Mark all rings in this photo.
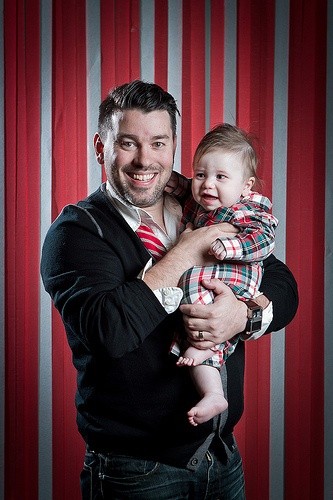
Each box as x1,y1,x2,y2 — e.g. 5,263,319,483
198,331,204,341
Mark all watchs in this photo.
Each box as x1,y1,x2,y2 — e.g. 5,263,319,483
243,301,262,334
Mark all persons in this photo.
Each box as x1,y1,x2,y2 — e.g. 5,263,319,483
164,123,280,426
40,80,298,500
